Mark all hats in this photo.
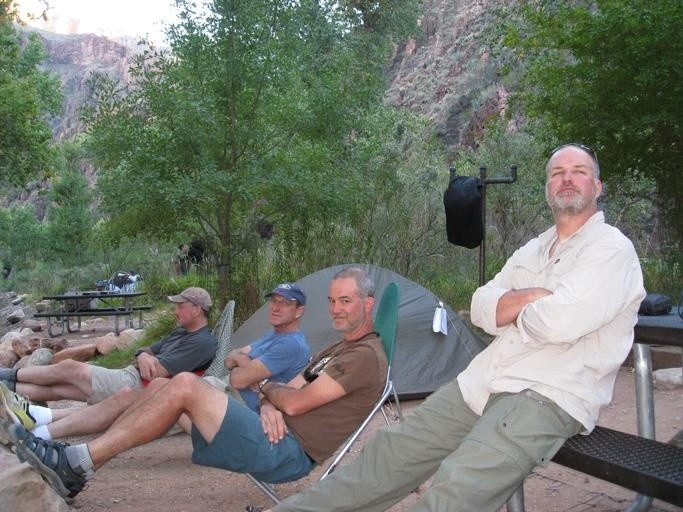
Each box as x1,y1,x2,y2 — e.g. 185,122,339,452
166,287,213,312
264,282,307,305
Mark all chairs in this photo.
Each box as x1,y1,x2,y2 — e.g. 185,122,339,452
243,282,404,504
200,299,235,380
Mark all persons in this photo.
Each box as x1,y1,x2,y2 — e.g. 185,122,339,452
1,286,219,408
127,270,141,290
174,239,204,277
260,144,648,512
0,281,311,443
8,268,387,506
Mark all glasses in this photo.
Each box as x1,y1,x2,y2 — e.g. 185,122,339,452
303,357,330,382
550,141,597,156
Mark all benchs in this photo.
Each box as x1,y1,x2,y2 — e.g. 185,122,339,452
32,305,152,338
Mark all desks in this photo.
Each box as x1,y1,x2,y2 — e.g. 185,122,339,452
41,291,146,311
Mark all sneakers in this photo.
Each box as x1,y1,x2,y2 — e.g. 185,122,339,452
0,382,37,433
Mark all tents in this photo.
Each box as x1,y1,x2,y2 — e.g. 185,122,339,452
231,263,490,403
110,270,131,291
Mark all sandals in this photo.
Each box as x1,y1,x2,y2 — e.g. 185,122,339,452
9,423,88,499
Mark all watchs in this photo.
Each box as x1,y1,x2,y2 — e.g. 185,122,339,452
257,378,271,390
